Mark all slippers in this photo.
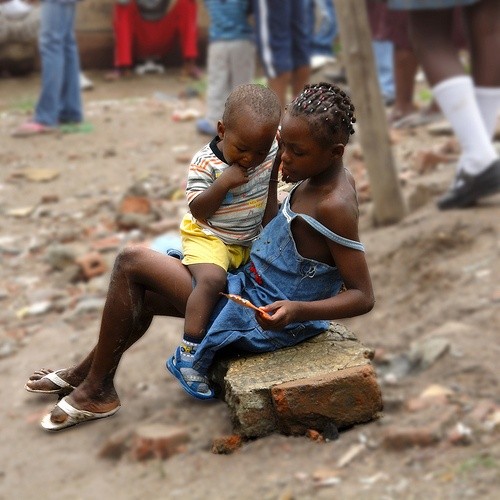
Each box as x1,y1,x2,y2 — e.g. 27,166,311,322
24,369,78,394
40,396,120,432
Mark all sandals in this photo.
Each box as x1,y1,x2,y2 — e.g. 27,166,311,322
167,346,214,399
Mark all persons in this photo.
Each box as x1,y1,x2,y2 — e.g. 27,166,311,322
389,0,500,215
381,0,456,128
308,1,336,70
23,83,376,431
253,0,311,130
167,85,282,399
17,0,82,137
366,1,396,104
107,0,202,84
0,0,94,92
197,0,255,134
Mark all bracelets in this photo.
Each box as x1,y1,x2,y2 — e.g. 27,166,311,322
269,178,279,184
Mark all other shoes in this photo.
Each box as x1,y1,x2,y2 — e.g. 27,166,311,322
79,73,93,90
15,123,55,137
325,68,347,86
435,160,500,210
197,121,214,134
